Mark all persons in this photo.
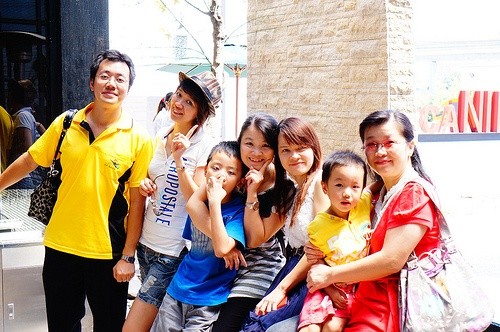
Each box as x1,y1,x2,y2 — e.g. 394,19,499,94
304,109,442,332
153,92,175,139
148,140,252,332
0,105,14,176
7,79,42,189
120,70,222,332
297,150,386,332
186,114,296,332
0,50,154,332
239,116,331,332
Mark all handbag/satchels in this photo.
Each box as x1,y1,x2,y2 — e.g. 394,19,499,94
396,250,496,332
27,109,78,225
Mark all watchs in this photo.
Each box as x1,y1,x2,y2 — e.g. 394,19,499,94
121,255,135,263
245,201,260,211
176,165,186,170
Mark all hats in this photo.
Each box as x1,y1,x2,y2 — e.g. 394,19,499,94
178,70,222,117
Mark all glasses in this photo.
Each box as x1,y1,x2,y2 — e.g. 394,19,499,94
360,141,398,153
97,73,126,85
149,175,174,216
